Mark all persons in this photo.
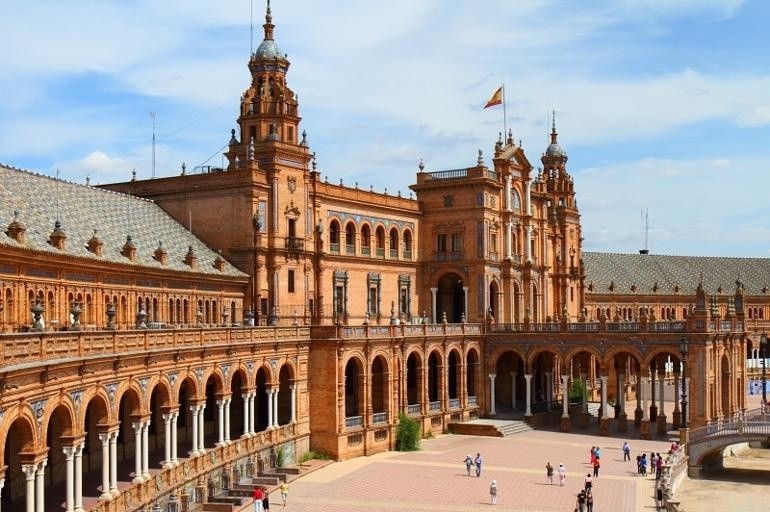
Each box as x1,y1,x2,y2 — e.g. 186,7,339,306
545,442,679,512
262,485,270,512
474,452,481,476
489,479,497,504
253,486,264,511
463,454,473,475
280,481,289,505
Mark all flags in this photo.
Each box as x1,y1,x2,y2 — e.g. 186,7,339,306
481,86,501,111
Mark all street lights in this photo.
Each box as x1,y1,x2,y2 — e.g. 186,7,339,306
677,336,690,445
760,328,768,400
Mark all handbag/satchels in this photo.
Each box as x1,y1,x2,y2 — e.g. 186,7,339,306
574,508,578,512
